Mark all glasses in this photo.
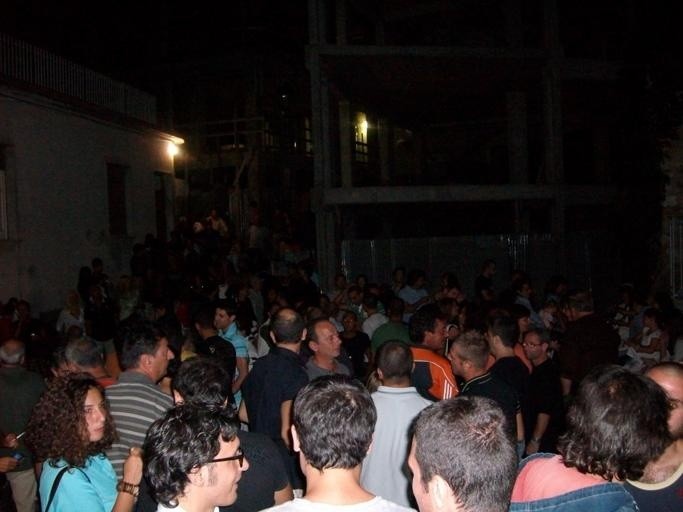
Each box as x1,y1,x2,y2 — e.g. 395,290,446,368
522,341,543,348
205,446,245,468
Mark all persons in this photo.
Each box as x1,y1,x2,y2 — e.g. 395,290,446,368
1,208,683,512
